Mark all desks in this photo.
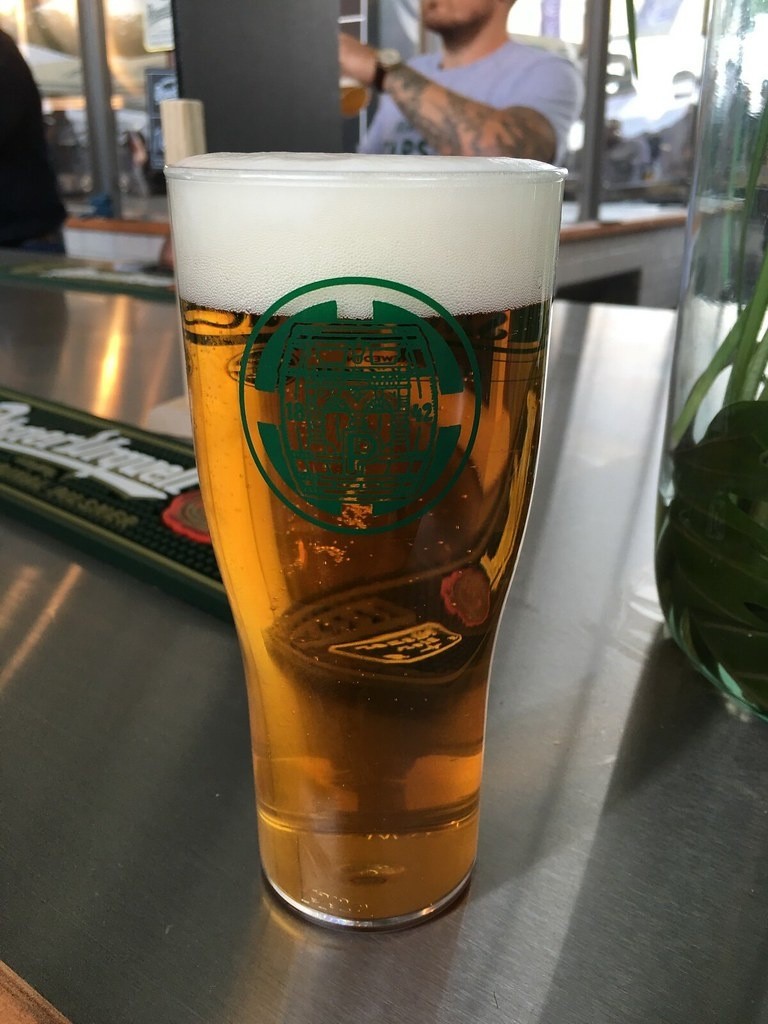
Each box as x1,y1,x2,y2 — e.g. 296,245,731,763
0,268,768,1022
58,192,693,301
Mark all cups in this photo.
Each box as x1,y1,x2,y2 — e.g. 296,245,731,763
166,143,565,935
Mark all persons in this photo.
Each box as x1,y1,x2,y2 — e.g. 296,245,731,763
338,0,588,168
0,29,73,260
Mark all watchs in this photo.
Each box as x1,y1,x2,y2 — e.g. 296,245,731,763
369,47,403,93
